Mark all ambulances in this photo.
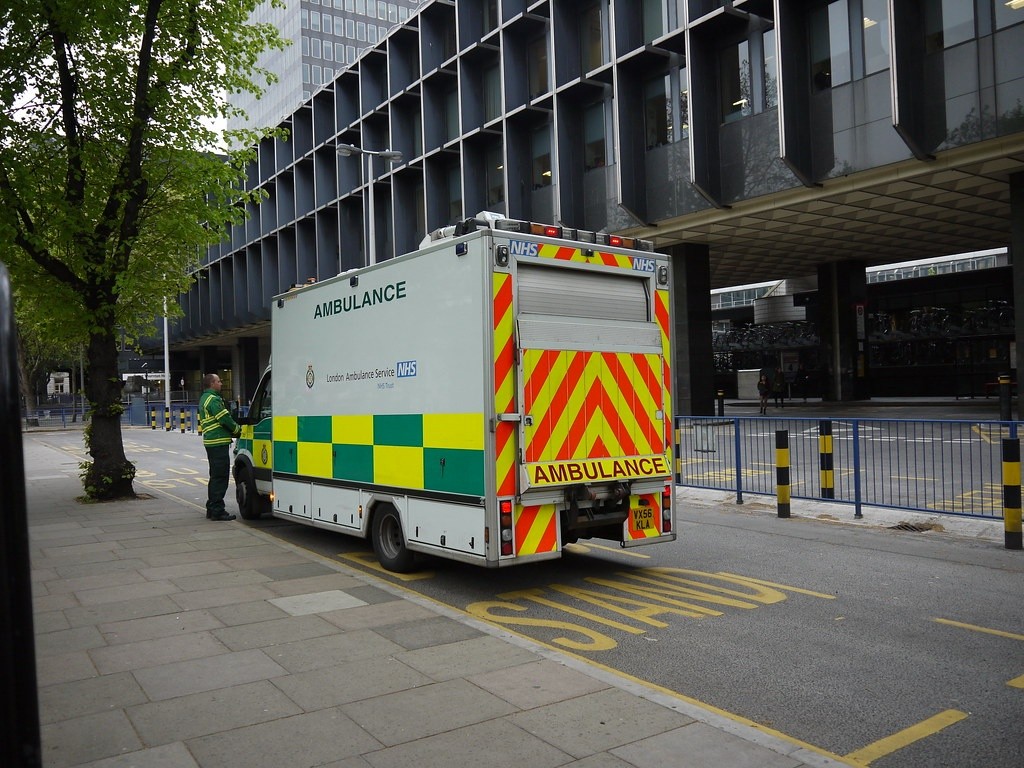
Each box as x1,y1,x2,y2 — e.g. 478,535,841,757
231,210,682,575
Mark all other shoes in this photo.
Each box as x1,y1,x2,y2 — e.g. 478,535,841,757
206,509,236,520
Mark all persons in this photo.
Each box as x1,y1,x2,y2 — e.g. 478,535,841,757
199,374,242,521
772,377,785,408
757,375,770,415
